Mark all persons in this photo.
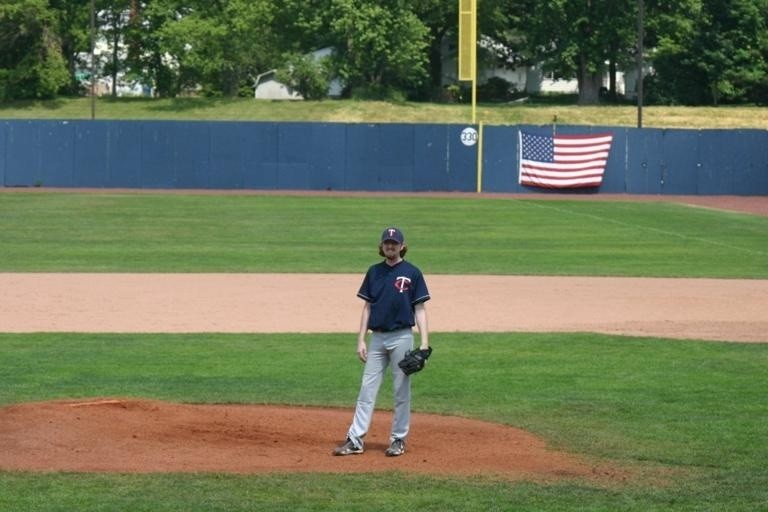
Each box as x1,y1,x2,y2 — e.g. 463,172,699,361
330,227,431,456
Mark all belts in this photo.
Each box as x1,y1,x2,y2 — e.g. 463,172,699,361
371,325,413,333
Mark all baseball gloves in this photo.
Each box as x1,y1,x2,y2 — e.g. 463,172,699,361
398,346,432,375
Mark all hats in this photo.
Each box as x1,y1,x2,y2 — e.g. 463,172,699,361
380,227,405,244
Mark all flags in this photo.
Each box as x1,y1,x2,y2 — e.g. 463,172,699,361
518,129,612,189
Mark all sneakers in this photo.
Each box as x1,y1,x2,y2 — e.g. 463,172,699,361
332,436,366,456
385,439,406,456
